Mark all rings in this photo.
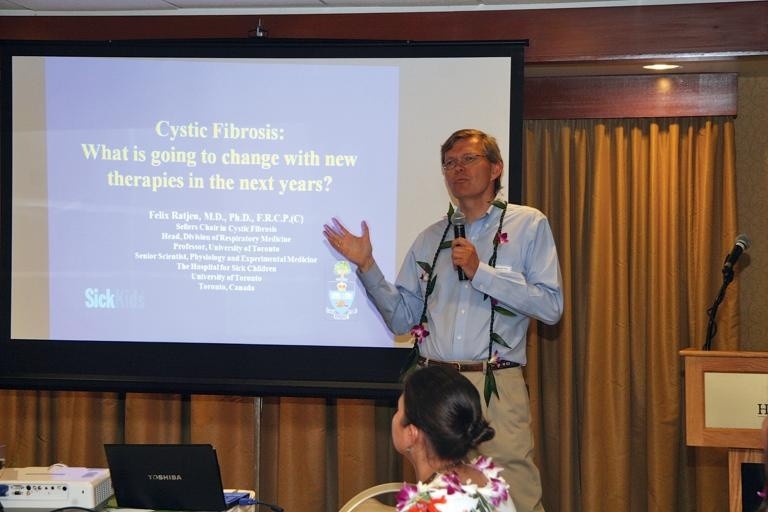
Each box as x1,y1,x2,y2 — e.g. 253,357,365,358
337,241,341,248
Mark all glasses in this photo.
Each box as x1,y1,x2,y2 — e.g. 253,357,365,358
441,154,487,170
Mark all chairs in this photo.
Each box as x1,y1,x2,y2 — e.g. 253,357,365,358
340,481,425,512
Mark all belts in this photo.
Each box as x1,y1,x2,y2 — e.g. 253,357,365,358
417,353,520,372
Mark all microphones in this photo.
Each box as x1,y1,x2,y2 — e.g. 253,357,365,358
723,232,751,274
451,211,470,280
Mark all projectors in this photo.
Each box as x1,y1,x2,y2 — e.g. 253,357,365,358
0,462,112,511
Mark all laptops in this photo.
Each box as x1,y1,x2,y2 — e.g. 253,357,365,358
105,440,250,511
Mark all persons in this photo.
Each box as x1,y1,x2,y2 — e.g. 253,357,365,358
390,363,518,512
321,127,565,512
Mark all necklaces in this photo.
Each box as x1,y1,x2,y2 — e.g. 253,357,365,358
423,461,461,484
